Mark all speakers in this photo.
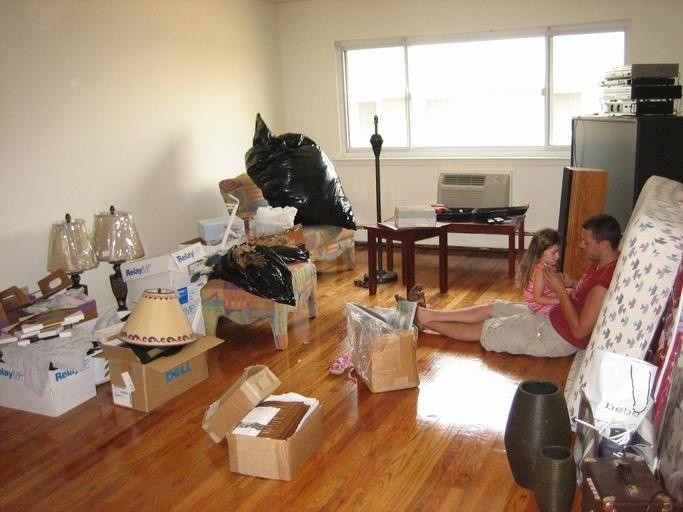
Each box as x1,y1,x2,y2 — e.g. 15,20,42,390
558,166,608,280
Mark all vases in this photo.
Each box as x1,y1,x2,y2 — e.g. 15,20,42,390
503,375,573,492
531,440,577,512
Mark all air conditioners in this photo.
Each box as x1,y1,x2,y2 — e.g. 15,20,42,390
436,172,511,209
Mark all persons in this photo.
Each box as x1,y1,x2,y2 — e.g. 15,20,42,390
513,227,578,313
393,213,620,359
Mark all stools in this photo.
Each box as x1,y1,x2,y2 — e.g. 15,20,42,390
194,261,318,349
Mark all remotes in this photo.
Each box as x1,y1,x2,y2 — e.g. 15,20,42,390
494,217,504,223
486,217,495,224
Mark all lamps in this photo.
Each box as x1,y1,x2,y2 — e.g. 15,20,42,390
90,204,146,313
42,211,99,294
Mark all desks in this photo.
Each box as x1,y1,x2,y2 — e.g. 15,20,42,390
383,208,526,279
362,220,453,299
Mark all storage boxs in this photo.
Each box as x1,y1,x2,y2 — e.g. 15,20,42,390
2,244,226,419
248,224,304,248
335,294,420,393
197,215,245,241
199,364,283,445
224,396,326,484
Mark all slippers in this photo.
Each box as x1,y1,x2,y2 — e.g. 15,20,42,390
393,294,427,333
408,283,427,310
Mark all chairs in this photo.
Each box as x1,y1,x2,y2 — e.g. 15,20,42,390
217,169,358,271
